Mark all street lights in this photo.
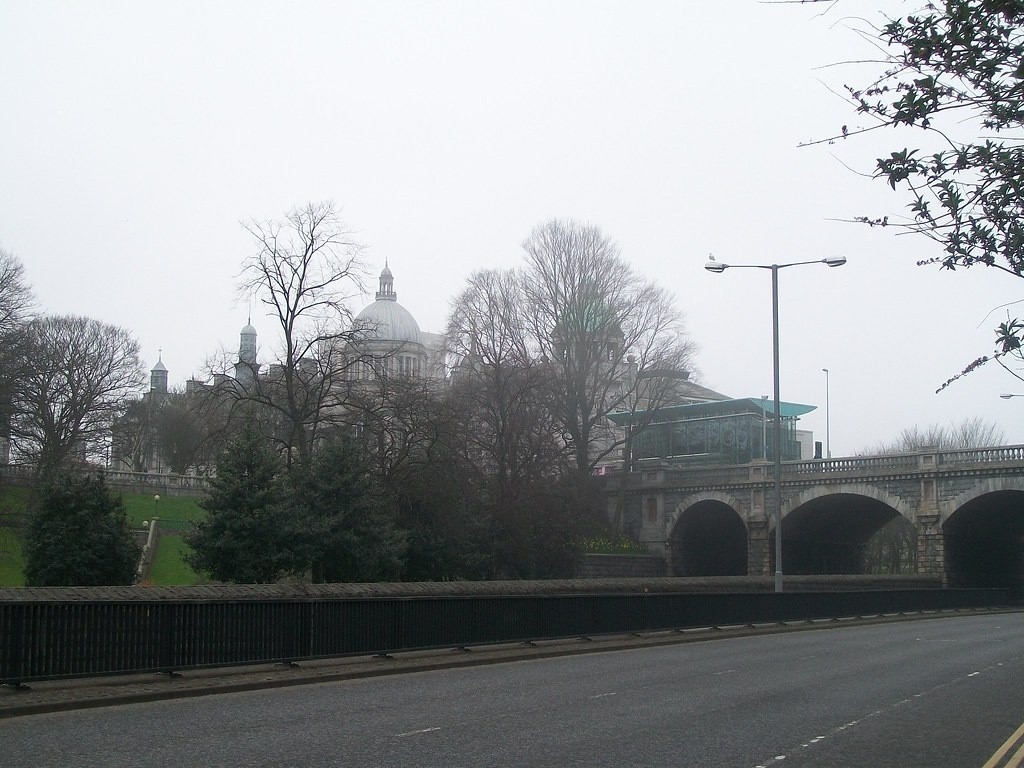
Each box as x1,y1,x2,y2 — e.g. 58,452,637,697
821,368,830,458
760,395,768,460
154,495,160,517
143,520,148,545
705,256,848,590
